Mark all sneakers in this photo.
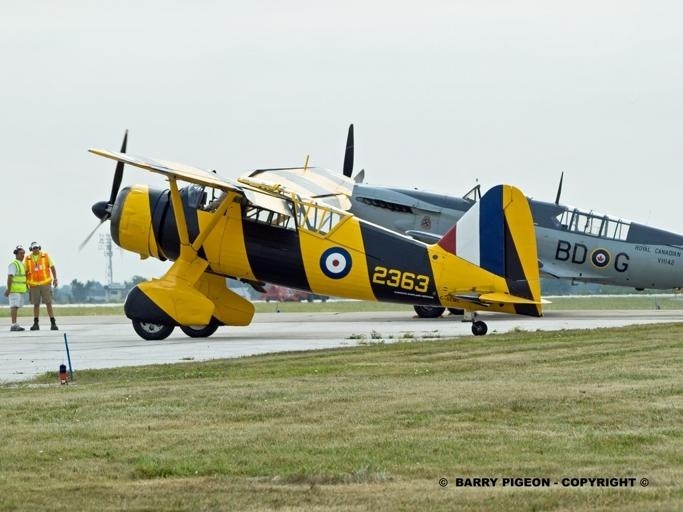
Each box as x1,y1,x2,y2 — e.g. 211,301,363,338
10,324,25,331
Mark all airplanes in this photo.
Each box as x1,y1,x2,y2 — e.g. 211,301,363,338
76,123,683,340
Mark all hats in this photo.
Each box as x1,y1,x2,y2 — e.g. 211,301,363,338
31,242,40,248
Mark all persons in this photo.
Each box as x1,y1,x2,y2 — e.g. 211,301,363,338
3,242,59,331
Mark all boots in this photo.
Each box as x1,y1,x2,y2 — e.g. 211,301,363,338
29,318,40,330
49,317,59,330
